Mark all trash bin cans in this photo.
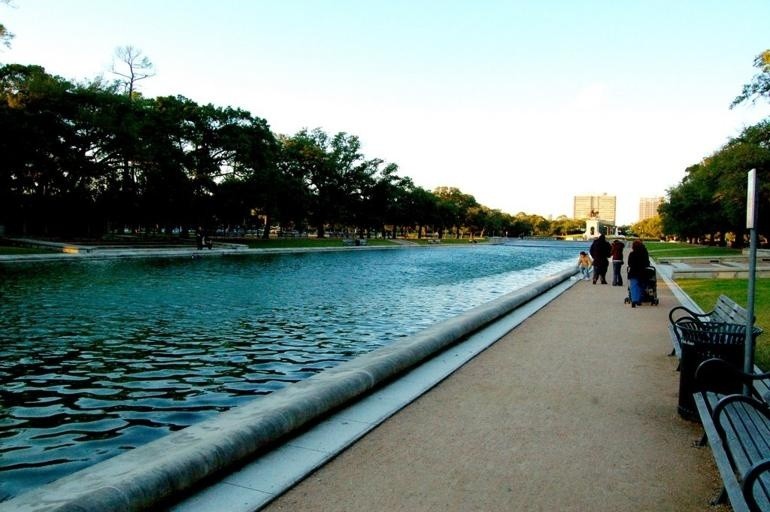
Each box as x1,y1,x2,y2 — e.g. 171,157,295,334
674,320,764,423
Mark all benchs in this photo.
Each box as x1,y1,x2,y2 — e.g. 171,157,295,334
667,291,770,511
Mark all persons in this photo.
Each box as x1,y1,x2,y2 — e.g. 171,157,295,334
590,235,611,284
196,224,206,249
626,240,649,307
612,239,624,287
577,252,593,281
714,231,767,248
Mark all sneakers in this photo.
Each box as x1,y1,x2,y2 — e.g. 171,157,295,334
618,282,622,285
612,282,617,286
632,301,641,308
593,278,597,284
601,281,607,284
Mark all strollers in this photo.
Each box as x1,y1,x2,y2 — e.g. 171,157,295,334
624,266,658,306
